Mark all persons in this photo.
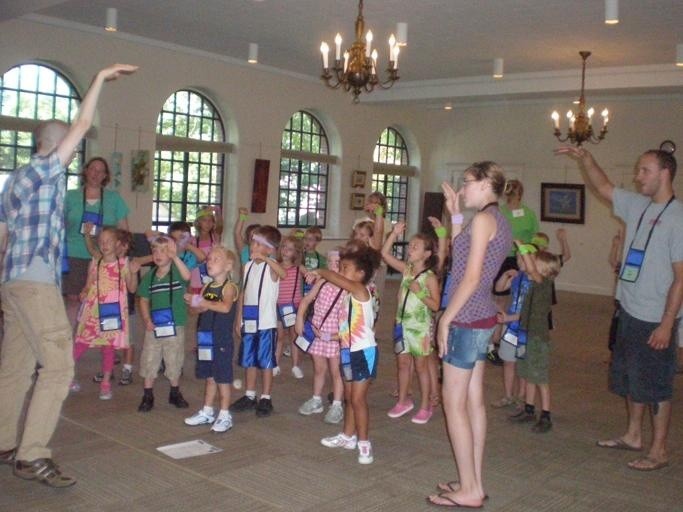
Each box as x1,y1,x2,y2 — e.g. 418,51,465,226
0,63,139,488
426,160,519,511
554,146,683,470
132,178,571,465
63,157,133,402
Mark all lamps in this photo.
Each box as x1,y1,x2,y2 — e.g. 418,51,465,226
491,58,504,79
676,44,682,66
104,7,117,31
246,43,257,64
549,51,611,146
318,1,403,105
394,23,407,48
603,0,618,25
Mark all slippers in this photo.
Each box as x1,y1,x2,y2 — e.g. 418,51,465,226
437,480,490,505
425,492,482,512
628,455,669,472
596,437,644,452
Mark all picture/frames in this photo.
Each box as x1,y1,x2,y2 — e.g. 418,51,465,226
540,182,584,225
351,170,366,188
351,192,364,209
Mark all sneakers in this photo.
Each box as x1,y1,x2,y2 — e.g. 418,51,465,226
13,457,74,488
93,355,190,412
298,386,442,465
492,394,553,432
185,367,303,433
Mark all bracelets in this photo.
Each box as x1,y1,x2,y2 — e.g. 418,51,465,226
451,213,464,226
